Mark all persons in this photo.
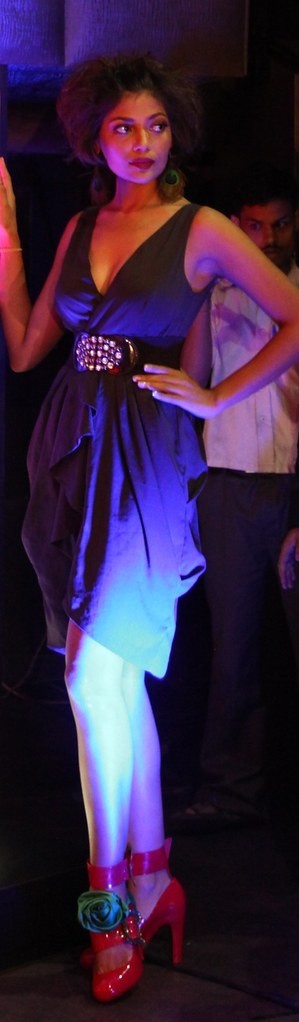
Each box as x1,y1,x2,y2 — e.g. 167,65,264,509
0,50,299,998
169,173,299,828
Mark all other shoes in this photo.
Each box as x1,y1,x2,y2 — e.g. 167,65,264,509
170,802,260,829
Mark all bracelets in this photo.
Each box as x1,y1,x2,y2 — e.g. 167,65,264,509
0,248,23,251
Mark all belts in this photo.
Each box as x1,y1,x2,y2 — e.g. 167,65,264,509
70,331,181,376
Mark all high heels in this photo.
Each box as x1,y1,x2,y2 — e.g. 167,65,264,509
80,837,186,972
86,854,144,1002
